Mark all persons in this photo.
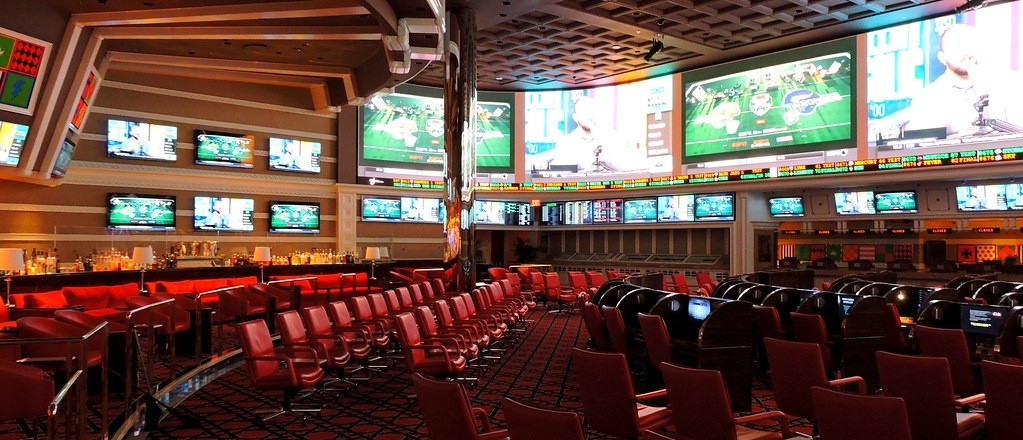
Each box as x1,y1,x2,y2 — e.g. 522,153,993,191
546,95,622,173
964,188,987,209
842,194,860,213
205,200,230,228
121,125,151,156
476,203,492,222
662,198,680,220
407,200,424,221
280,142,301,169
906,23,1023,137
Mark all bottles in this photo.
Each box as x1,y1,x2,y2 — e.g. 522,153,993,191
22,247,360,277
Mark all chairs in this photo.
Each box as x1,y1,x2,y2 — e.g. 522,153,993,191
0,262,1023,439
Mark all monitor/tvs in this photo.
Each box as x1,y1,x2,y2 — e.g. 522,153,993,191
833,191,919,215
106,119,178,163
105,192,176,233
688,298,712,321
959,303,1009,338
193,129,255,169
268,137,322,174
956,183,1023,212
193,197,255,233
769,197,804,217
359,193,534,227
539,191,736,227
268,200,321,234
841,294,863,314
